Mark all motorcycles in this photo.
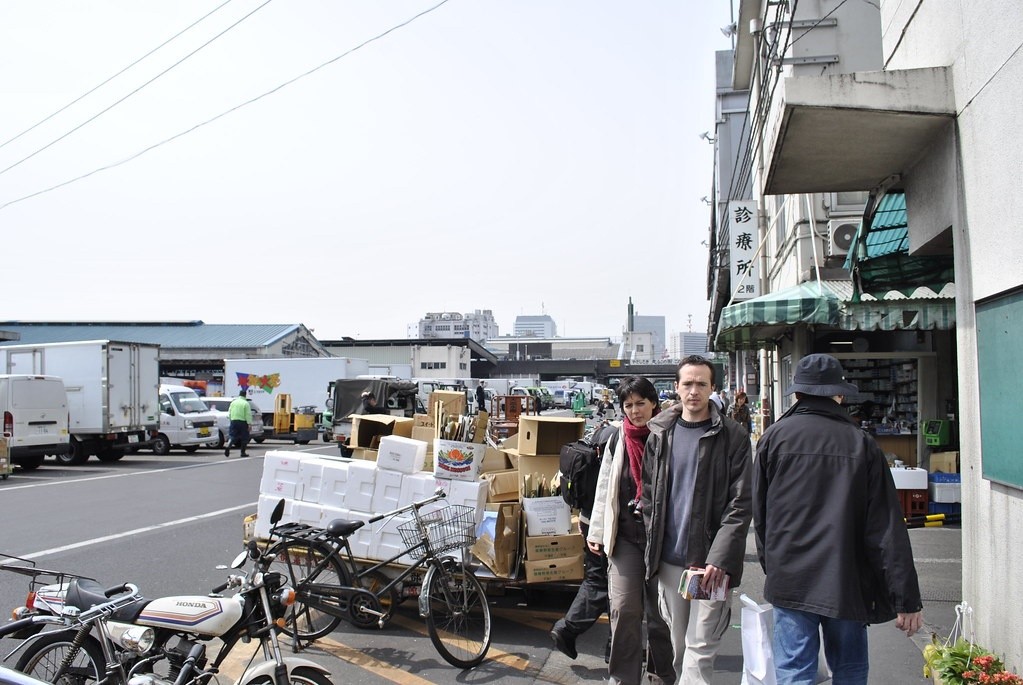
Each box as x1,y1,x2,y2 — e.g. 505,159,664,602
488,396,540,445
0,497,342,685
597,409,617,420
239,514,732,640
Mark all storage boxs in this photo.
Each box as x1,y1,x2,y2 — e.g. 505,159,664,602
890,451,961,529
0,437,9,474
255,389,587,584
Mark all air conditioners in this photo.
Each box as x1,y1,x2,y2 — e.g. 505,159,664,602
828,219,862,256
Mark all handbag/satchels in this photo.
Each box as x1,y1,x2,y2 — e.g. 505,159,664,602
740,593,833,685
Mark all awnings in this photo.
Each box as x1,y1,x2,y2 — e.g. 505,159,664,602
714,281,956,353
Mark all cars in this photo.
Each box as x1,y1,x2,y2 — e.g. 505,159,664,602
658,390,674,401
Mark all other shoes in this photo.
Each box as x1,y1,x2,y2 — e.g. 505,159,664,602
605,640,646,663
552,629,578,660
241,453,249,457
225,447,230,457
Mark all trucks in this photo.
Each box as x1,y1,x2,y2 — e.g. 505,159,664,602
222,359,618,458
0,340,161,465
0,374,70,472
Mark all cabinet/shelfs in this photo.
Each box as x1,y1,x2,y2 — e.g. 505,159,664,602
839,359,918,435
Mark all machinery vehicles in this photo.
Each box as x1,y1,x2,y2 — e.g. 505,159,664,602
253,394,319,446
322,381,336,442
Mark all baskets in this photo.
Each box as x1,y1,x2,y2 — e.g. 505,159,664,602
396,504,476,560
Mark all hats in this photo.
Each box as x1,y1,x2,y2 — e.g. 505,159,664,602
783,354,858,397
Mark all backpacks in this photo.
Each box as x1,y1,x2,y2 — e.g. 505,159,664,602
560,425,613,509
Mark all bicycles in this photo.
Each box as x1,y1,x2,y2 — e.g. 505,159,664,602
0,581,143,685
255,487,491,669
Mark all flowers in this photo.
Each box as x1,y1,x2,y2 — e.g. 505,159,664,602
922,631,1023,685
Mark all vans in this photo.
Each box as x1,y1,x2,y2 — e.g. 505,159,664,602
162,398,266,449
127,384,220,455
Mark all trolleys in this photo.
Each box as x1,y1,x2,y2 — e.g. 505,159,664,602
574,407,594,420
0,432,11,479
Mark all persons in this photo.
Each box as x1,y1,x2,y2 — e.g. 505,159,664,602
586,378,675,685
361,391,386,414
224,390,252,457
576,388,586,410
476,381,485,411
709,385,752,438
640,355,753,685
536,394,542,416
752,353,923,685
551,419,647,663
597,395,617,420
